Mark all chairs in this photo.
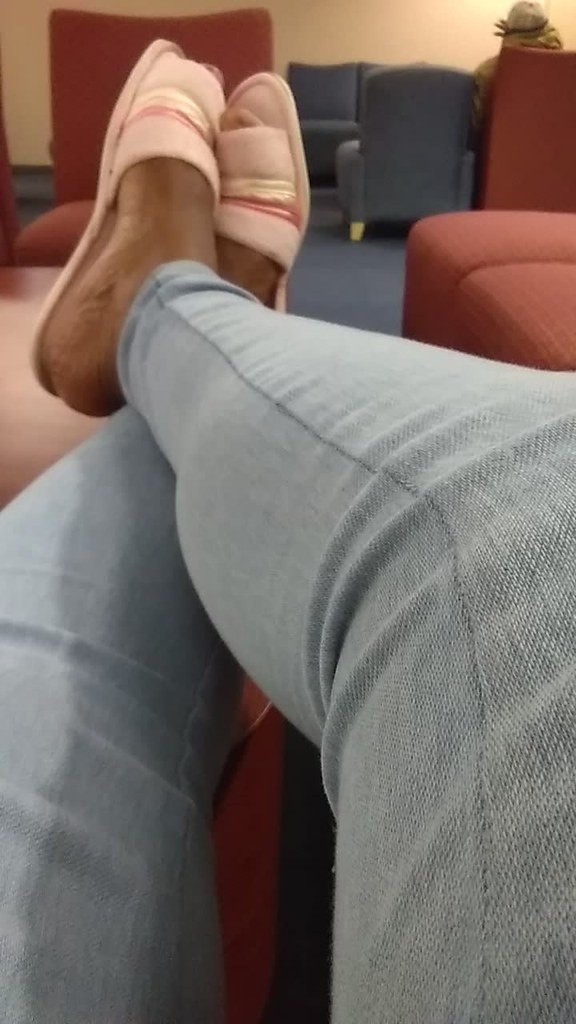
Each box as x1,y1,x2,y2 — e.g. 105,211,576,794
0,9,576,1024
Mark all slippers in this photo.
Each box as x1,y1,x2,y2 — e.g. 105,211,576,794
30,39,309,396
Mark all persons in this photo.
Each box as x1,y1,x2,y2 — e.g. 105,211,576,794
471,1,567,124
0,38,576,1024
